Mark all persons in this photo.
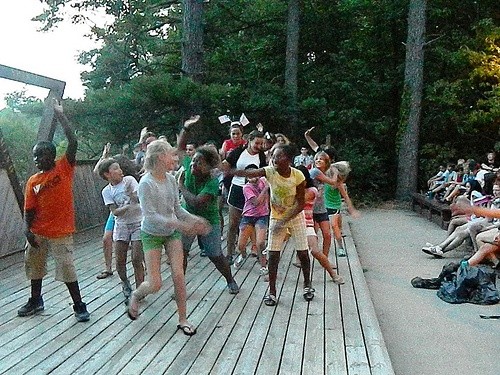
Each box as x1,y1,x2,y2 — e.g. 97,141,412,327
422,143,500,269
128,140,210,336
93,115,360,307
17,98,91,323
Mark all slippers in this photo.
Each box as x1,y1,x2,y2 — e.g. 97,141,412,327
128,290,138,320
96,271,113,279
177,324,196,335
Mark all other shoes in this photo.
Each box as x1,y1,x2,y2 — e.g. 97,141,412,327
73,302,90,322
234,252,248,269
260,267,268,276
263,291,277,306
421,243,443,258
122,280,131,297
18,295,44,316
338,248,346,257
330,276,345,284
227,280,239,294
200,250,206,257
303,286,315,301
225,255,234,265
292,263,302,268
